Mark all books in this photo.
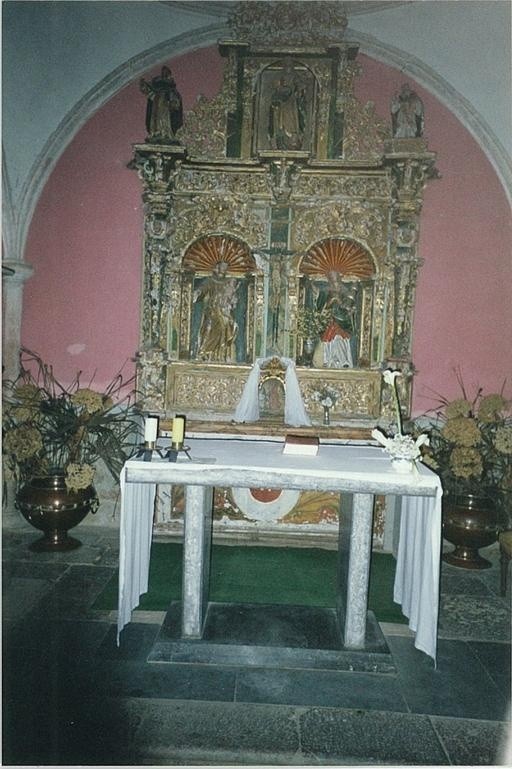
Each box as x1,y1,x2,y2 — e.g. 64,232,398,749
283,435,319,455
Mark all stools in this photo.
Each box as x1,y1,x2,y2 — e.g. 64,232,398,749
495,526,512,597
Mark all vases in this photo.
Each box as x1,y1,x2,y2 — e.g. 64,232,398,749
388,452,414,476
323,405,331,426
440,488,498,570
12,468,102,553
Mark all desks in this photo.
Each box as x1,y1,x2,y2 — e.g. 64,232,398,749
120,434,443,655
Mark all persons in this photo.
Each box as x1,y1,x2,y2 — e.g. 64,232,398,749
312,269,355,368
391,83,425,139
191,262,239,365
139,67,182,145
268,73,306,150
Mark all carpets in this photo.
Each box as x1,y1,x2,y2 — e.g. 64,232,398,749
83,537,446,631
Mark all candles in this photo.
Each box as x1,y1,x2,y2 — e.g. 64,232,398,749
171,417,185,444
143,417,158,443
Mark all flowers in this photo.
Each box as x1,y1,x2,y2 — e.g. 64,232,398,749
303,376,352,411
367,367,431,467
2,339,160,523
400,360,511,502
